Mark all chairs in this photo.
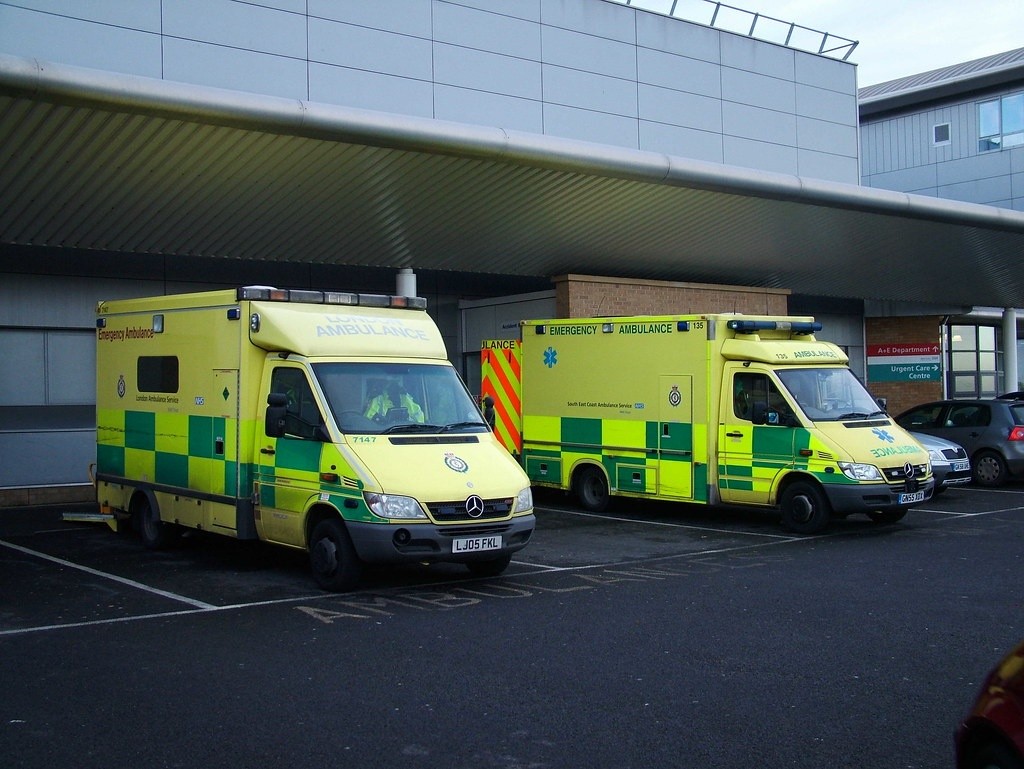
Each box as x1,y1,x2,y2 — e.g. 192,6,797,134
954,414,966,424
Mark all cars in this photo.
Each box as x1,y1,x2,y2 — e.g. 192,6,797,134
891,397,1024,489
967,391,1024,426
904,429,973,494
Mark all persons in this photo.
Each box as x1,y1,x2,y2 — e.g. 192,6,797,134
364,379,424,423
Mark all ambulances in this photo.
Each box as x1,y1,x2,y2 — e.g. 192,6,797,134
85,284,538,592
477,310,935,537
